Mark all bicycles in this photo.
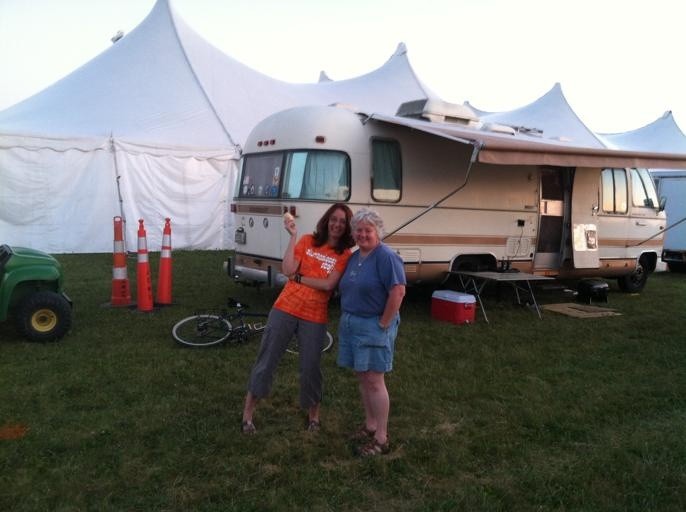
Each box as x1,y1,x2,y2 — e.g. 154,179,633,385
171,298,335,358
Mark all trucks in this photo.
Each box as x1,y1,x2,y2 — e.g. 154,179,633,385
647,169,685,269
224,99,671,303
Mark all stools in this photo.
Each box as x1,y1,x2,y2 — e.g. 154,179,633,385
578,278,609,305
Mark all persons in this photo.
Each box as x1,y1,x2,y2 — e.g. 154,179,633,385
241,202,354,437
336,208,408,457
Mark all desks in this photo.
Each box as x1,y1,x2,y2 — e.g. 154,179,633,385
443,269,556,324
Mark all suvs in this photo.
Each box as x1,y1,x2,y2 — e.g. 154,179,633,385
0,244,75,345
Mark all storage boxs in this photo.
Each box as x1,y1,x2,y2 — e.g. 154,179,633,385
431,290,476,324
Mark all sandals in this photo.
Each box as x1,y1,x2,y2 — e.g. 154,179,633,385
360,434,391,462
240,416,259,440
306,417,322,437
348,424,377,444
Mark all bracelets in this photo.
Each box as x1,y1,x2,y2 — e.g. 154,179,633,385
294,273,302,283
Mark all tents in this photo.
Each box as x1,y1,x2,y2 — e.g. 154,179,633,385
0,0,685,253
